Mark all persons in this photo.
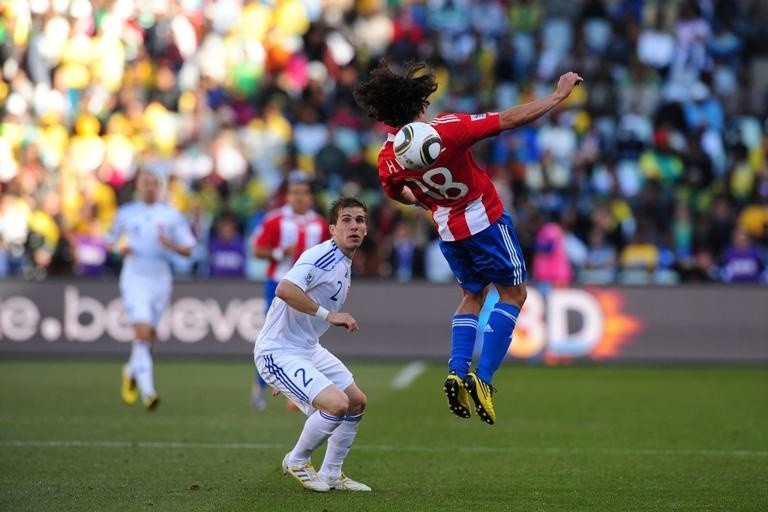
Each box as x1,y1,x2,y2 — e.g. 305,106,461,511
253,197,371,495
0,0,767,284
252,172,330,411
106,166,198,408
353,57,584,425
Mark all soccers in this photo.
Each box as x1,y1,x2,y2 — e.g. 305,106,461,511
393,122,442,169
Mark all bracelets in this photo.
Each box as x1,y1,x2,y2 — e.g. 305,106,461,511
315,305,330,320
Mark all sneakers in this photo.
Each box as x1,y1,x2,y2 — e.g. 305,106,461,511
318,472,371,492
443,371,472,418
121,366,158,409
282,452,329,492
462,370,495,424
